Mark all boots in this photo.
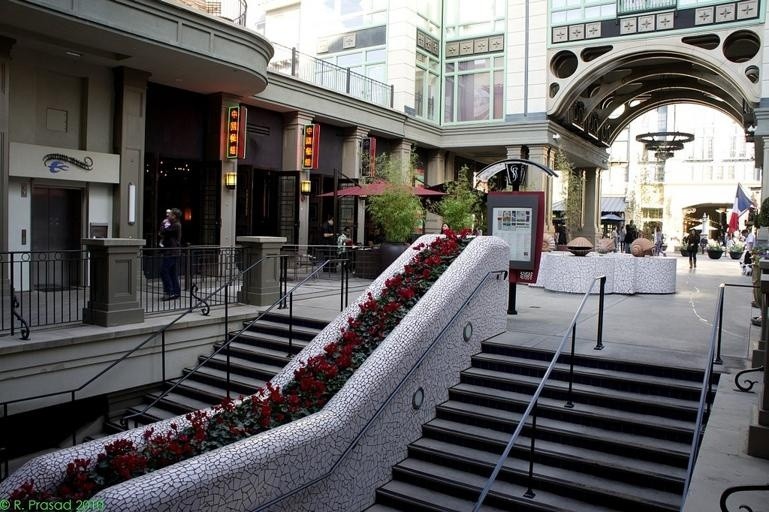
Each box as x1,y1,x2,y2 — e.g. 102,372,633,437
689,260,697,268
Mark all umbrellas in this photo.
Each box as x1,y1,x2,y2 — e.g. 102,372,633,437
315,179,450,200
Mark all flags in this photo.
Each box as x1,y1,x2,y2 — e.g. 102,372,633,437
728,185,753,233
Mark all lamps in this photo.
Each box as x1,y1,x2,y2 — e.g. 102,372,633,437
225,171,236,190
300,180,312,195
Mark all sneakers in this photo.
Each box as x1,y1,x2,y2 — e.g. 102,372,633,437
160,294,180,301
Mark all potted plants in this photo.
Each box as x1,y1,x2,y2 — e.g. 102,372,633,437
356,144,428,277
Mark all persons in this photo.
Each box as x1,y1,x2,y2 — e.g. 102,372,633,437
441,223,450,234
337,226,358,275
320,216,341,272
158,209,172,248
368,226,385,246
159,207,184,302
557,218,757,276
